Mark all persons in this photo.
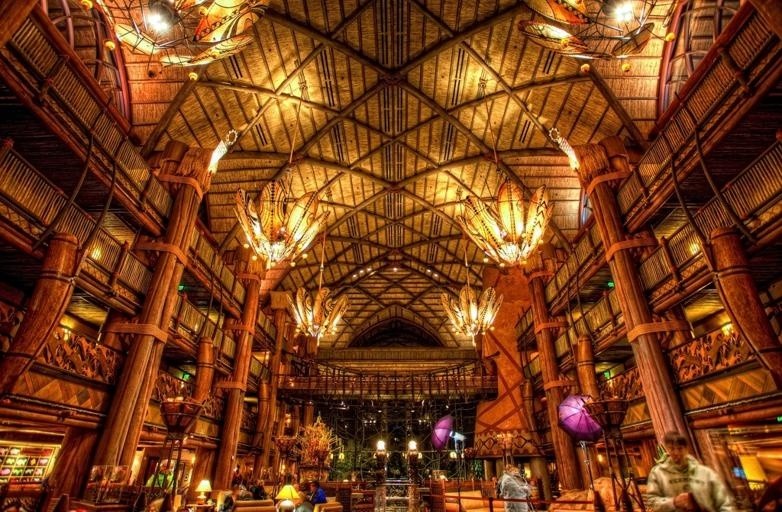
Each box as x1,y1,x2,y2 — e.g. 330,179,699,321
646,432,735,512
215,480,327,512
500,463,531,512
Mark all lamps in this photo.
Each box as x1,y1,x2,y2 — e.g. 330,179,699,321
432,414,469,512
516,1,661,66
229,81,330,273
455,65,557,268
194,478,214,503
274,484,300,511
437,183,505,339
557,394,606,494
80,0,270,86
284,222,355,344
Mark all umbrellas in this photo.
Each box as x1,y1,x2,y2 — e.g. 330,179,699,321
431,415,453,450
557,395,602,444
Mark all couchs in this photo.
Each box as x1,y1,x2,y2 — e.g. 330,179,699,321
305,487,353,511
217,489,276,512
